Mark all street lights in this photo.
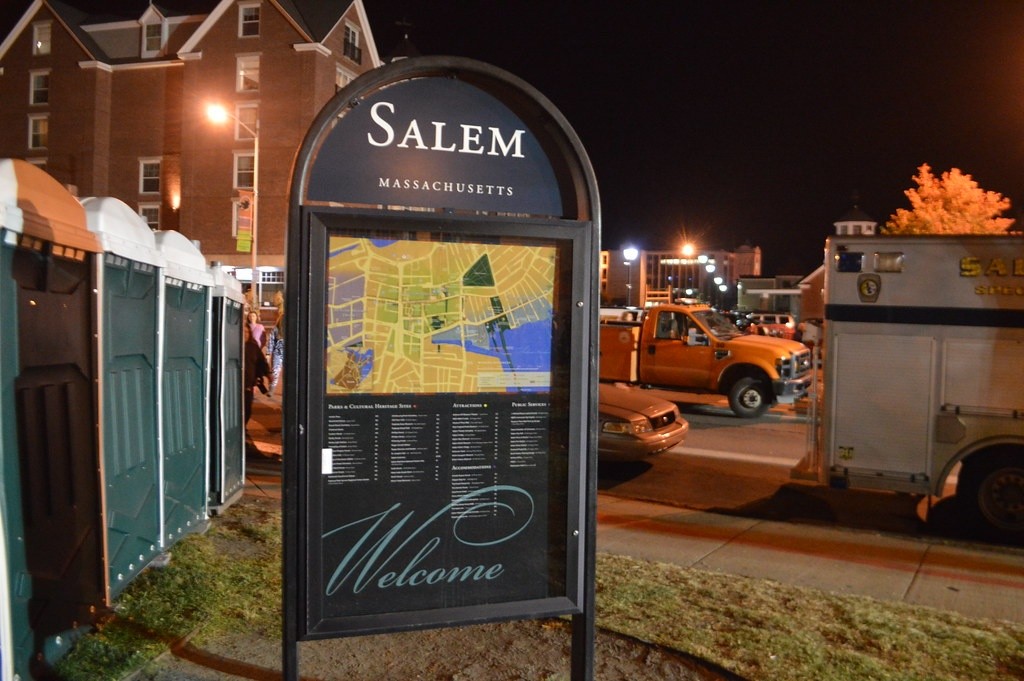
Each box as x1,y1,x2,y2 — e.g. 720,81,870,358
207,104,259,312
683,243,693,298
698,254,728,309
623,248,639,306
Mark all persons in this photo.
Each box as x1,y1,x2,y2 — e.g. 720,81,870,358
264,314,284,397
244,324,270,435
245,311,267,350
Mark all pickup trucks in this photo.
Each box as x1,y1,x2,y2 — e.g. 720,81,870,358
601,302,813,418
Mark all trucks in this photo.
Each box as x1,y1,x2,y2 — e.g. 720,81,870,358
785,234,1024,540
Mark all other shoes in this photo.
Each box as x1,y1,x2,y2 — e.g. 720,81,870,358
267,388,273,397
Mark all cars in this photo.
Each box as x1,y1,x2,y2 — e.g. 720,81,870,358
596,381,689,460
736,314,798,341
